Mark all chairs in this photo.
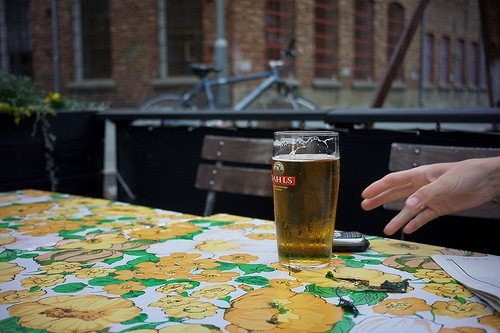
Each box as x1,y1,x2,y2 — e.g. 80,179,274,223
383,142,500,256
193,135,274,217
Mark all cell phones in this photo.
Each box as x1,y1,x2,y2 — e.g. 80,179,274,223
331,231,365,253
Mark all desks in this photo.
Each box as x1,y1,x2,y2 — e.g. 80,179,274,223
1,189,500,333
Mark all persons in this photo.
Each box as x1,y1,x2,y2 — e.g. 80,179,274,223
360,154,500,236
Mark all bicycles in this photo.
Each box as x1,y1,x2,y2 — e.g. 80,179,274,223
137,36,325,126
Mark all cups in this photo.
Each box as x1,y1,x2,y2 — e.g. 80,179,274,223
271,130,340,271
